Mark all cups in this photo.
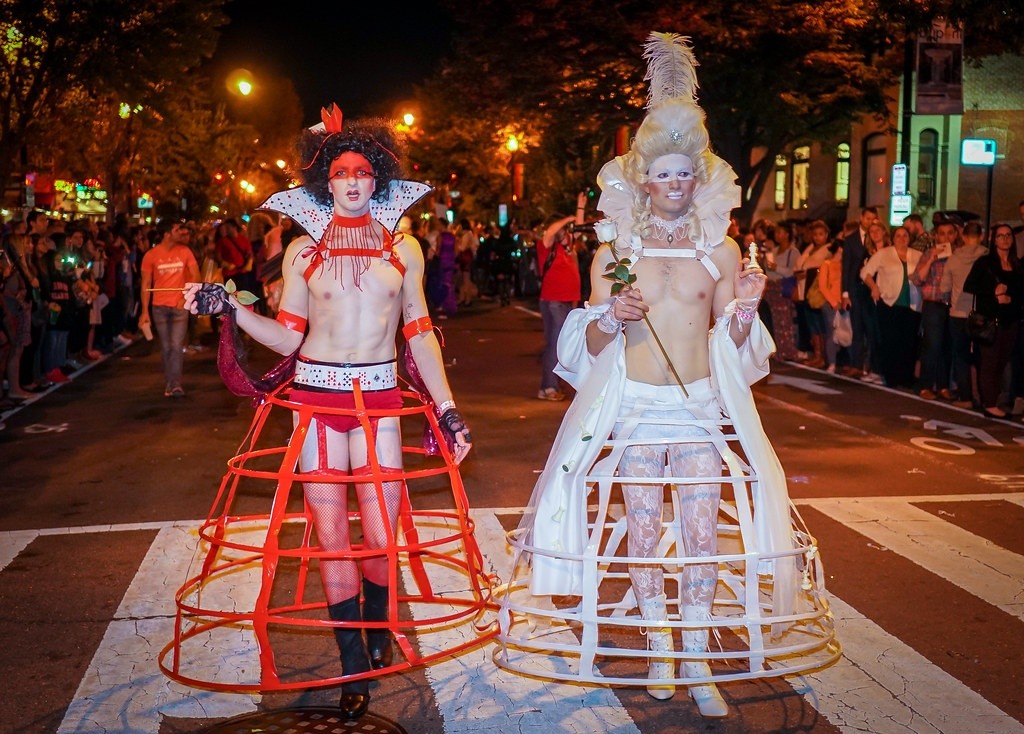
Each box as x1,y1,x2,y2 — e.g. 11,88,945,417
142,322,153,341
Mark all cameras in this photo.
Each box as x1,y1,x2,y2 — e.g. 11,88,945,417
569,221,596,233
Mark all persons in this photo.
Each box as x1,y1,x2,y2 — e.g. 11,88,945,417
0,187,1024,427
182,124,472,720
556,101,768,718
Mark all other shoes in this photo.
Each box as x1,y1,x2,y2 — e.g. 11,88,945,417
0,332,133,430
165,389,185,397
781,348,1024,423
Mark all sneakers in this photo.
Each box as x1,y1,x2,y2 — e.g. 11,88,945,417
538,388,566,401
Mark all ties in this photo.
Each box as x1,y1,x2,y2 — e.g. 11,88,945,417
864,233,867,246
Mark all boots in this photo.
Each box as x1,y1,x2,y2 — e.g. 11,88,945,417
640,592,676,700
679,603,730,716
362,576,393,670
327,592,371,721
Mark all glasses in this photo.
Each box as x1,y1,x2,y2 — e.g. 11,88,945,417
996,232,1013,240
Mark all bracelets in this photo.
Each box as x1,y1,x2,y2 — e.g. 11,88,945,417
438,400,456,415
576,207,585,210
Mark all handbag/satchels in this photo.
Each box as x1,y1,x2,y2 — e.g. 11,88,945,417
833,308,852,346
967,310,998,338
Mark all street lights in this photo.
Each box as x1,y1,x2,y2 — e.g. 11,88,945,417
507,135,519,207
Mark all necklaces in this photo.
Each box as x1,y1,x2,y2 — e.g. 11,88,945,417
648,214,690,245
321,211,382,292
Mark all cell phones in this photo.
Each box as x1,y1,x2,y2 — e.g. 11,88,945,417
87,261,92,269
